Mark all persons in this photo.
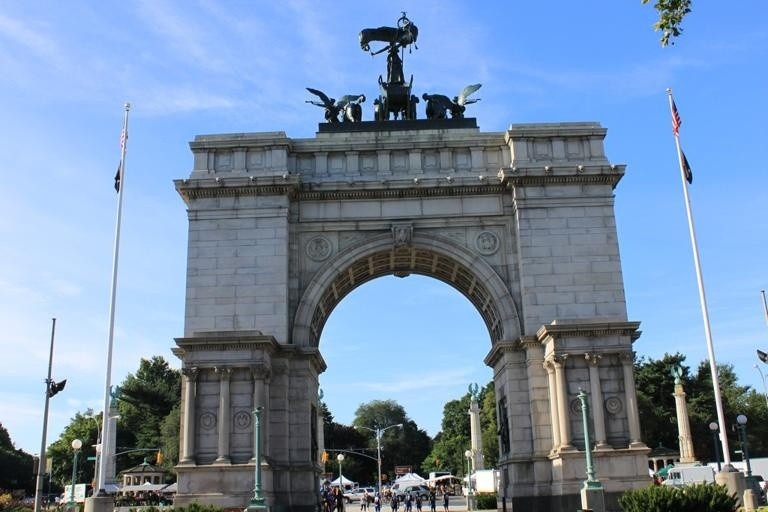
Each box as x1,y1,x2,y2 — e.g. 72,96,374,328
113,491,161,506
41,496,60,510
651,474,664,485
320,480,450,512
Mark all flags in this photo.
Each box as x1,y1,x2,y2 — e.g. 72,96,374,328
682,149,693,184
671,98,682,135
119,115,128,155
114,162,122,193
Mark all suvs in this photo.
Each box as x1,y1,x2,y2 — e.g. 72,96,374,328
395,484,431,501
340,487,376,503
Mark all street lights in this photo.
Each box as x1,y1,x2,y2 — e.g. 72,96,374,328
464,449,476,511
751,364,768,395
338,453,346,511
735,414,761,503
708,421,723,472
576,385,606,511
85,413,123,494
65,438,83,510
353,423,404,511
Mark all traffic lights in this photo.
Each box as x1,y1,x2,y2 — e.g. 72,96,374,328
157,452,164,463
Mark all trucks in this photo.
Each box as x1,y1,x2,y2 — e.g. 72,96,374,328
661,465,716,487
60,482,93,506
